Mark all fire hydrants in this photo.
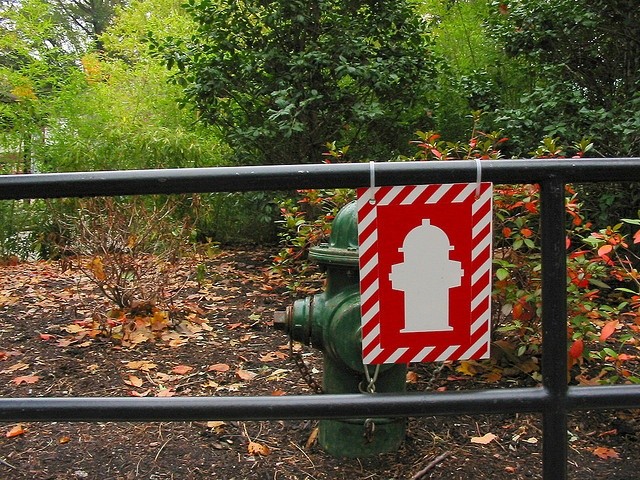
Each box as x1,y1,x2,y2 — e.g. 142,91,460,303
272,201,407,461
